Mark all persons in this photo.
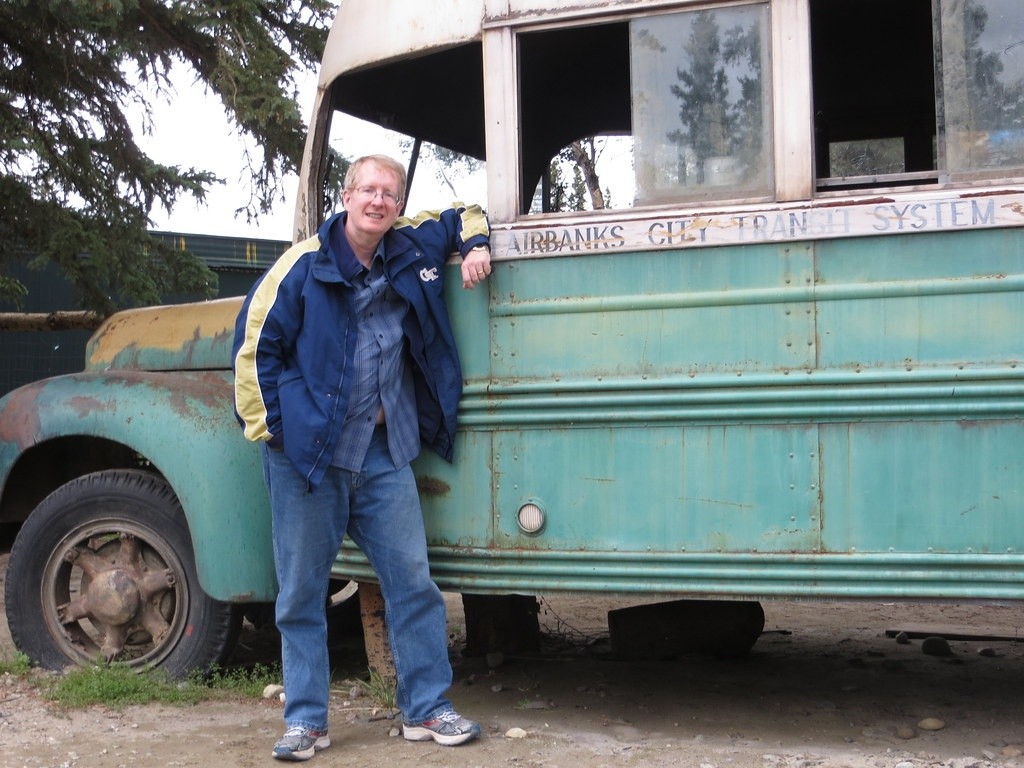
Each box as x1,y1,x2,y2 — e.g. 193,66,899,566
233,154,493,760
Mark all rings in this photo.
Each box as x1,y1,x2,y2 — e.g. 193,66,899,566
479,271,484,274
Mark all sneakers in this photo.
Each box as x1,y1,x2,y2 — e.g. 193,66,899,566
402,708,481,745
272,726,331,760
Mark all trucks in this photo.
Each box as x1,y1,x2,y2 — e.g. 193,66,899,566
0,0,1022,691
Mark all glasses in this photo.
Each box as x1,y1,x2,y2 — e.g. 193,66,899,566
353,186,401,207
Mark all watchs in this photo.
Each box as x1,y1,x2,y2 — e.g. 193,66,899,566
472,244,489,251
